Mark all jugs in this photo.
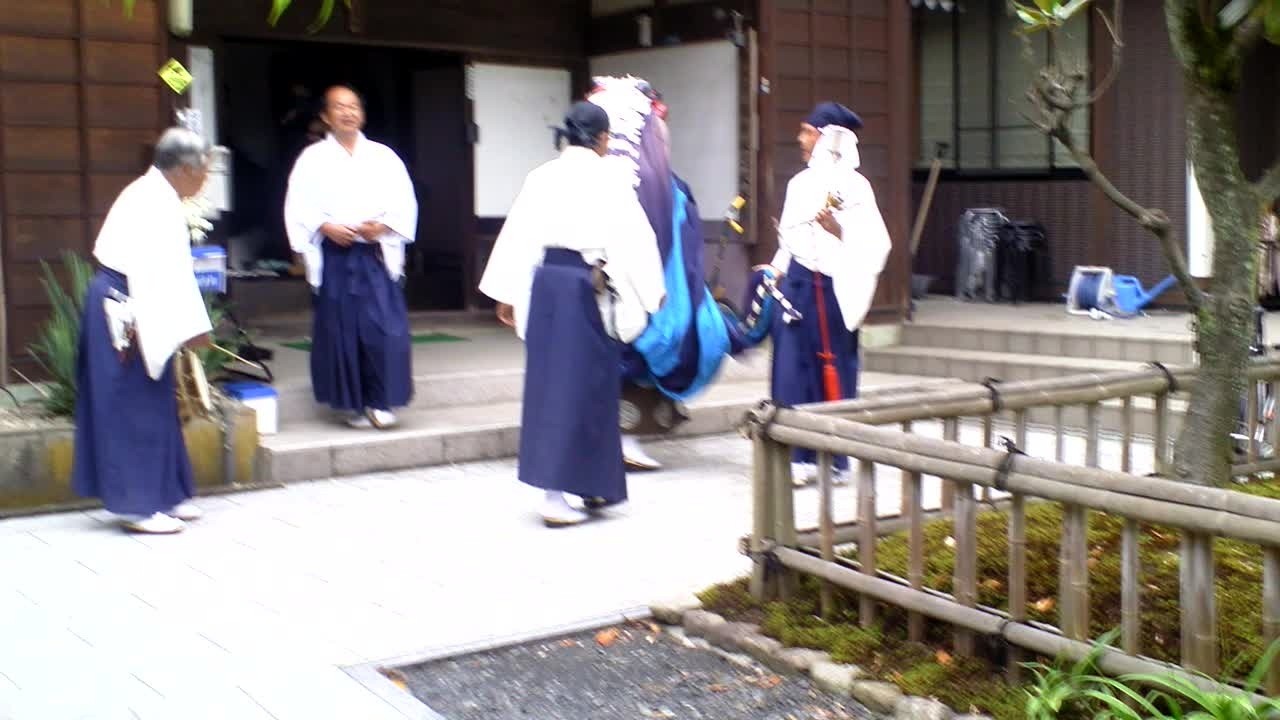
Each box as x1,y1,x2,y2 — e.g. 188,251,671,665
1112,274,1177,313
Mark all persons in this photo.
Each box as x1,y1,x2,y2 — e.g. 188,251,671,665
478,73,689,528
750,100,893,487
283,85,418,432
70,127,212,536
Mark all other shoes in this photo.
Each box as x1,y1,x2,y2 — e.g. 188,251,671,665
364,403,397,428
120,511,186,535
338,408,375,428
168,501,202,522
534,496,587,526
620,447,662,470
792,459,852,488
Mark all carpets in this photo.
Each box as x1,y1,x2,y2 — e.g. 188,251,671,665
281,335,469,352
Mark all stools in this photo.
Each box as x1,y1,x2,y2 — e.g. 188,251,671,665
958,207,1051,305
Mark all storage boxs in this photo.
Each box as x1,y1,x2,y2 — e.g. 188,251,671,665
189,243,226,293
224,382,277,433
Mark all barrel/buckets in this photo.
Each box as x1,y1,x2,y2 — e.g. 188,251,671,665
957,208,1038,301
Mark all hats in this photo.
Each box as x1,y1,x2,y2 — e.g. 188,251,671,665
803,100,863,132
